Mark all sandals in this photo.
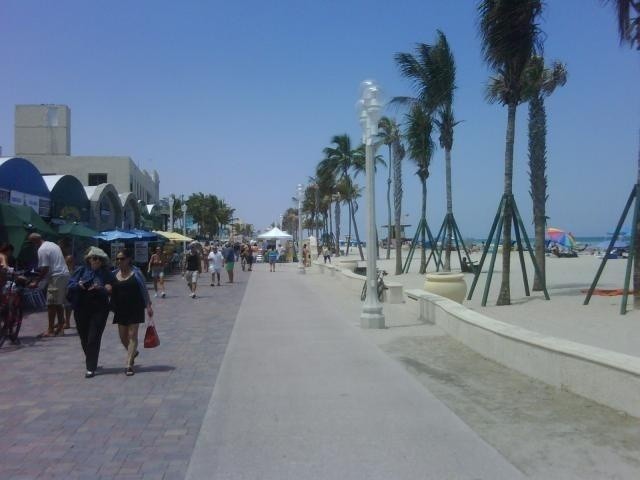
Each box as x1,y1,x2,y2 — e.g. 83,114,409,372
126,367,134,376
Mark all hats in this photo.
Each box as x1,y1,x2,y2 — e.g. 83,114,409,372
83,246,110,264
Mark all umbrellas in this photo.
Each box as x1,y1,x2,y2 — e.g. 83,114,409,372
547,227,578,252
598,239,630,251
91,227,138,268
124,227,160,268
622,230,633,240
148,232,170,243
152,230,194,242
51,218,107,266
607,224,632,235
256,225,294,240
0,199,63,260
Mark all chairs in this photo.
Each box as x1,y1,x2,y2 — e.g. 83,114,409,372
22,279,47,309
256,255,264,263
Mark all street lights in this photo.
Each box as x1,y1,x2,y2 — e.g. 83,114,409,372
297,182,308,274
168,197,175,232
279,213,283,231
181,204,188,275
353,77,386,328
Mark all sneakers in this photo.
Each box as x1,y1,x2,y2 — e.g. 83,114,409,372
85,370,94,377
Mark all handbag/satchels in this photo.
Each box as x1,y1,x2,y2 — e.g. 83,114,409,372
145,325,160,348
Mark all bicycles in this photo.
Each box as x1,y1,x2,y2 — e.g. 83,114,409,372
359,265,388,303
0,268,41,348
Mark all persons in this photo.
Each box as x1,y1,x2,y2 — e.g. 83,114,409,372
460,256,481,271
53,254,77,330
104,248,154,377
24,229,70,337
145,238,333,299
549,242,566,257
0,241,20,279
66,246,112,378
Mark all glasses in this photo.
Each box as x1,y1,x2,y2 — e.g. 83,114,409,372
92,256,103,260
